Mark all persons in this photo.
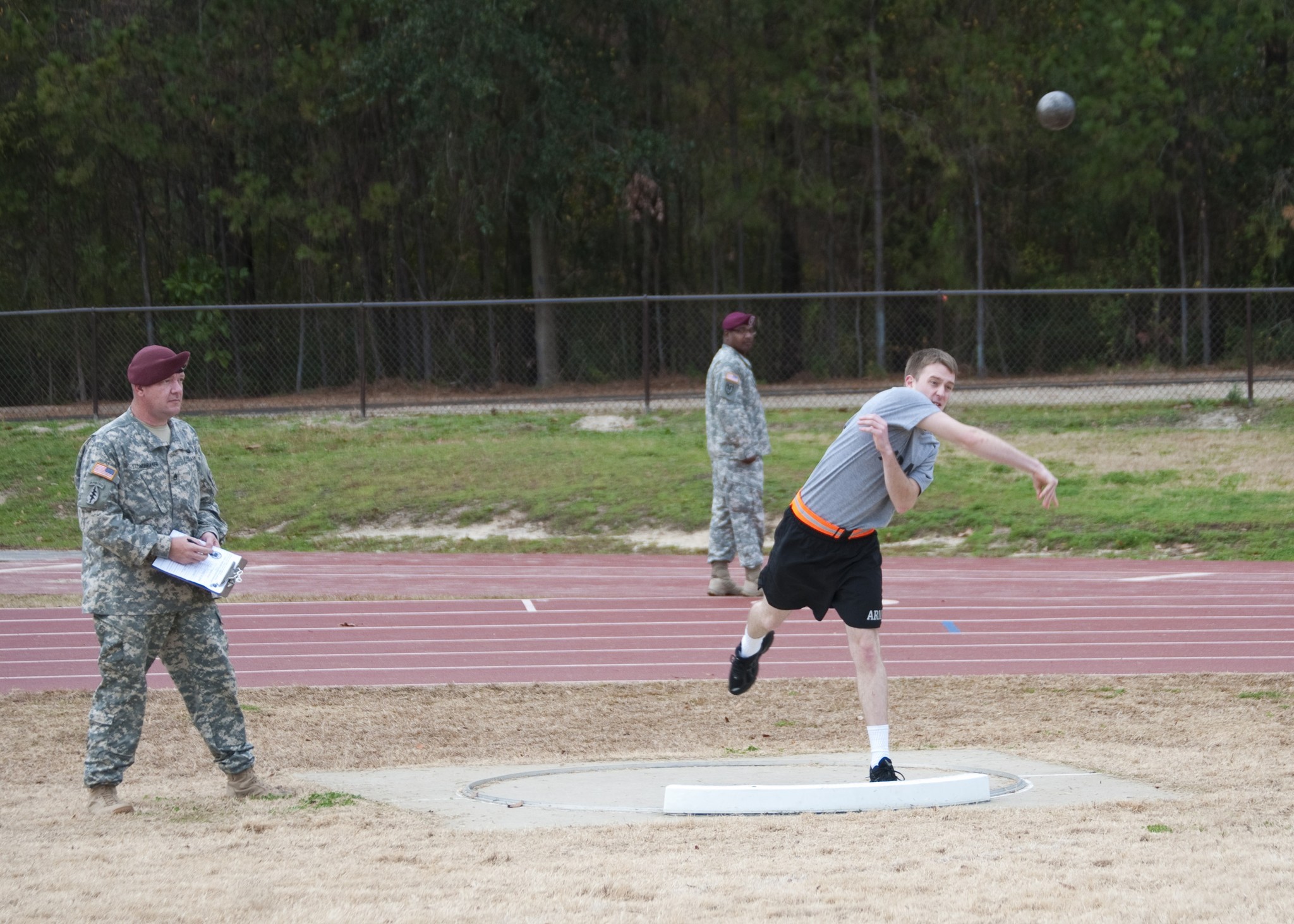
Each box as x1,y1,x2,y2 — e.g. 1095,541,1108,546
706,311,768,595
726,348,1060,781
75,345,304,813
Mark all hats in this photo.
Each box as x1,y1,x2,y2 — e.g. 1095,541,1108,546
127,345,190,385
723,312,756,330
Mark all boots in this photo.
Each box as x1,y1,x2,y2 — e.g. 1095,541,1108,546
87,785,134,816
741,564,764,596
707,562,742,596
226,766,280,801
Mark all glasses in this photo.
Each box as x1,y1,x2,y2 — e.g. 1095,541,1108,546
732,327,756,335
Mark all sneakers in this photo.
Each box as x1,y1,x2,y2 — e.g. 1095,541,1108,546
865,757,905,783
729,630,774,694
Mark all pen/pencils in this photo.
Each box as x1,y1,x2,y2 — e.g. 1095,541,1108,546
187,539,218,557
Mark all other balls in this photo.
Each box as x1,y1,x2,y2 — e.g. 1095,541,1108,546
1036,89,1074,130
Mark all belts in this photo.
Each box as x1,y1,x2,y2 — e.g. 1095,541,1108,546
789,489,875,540
